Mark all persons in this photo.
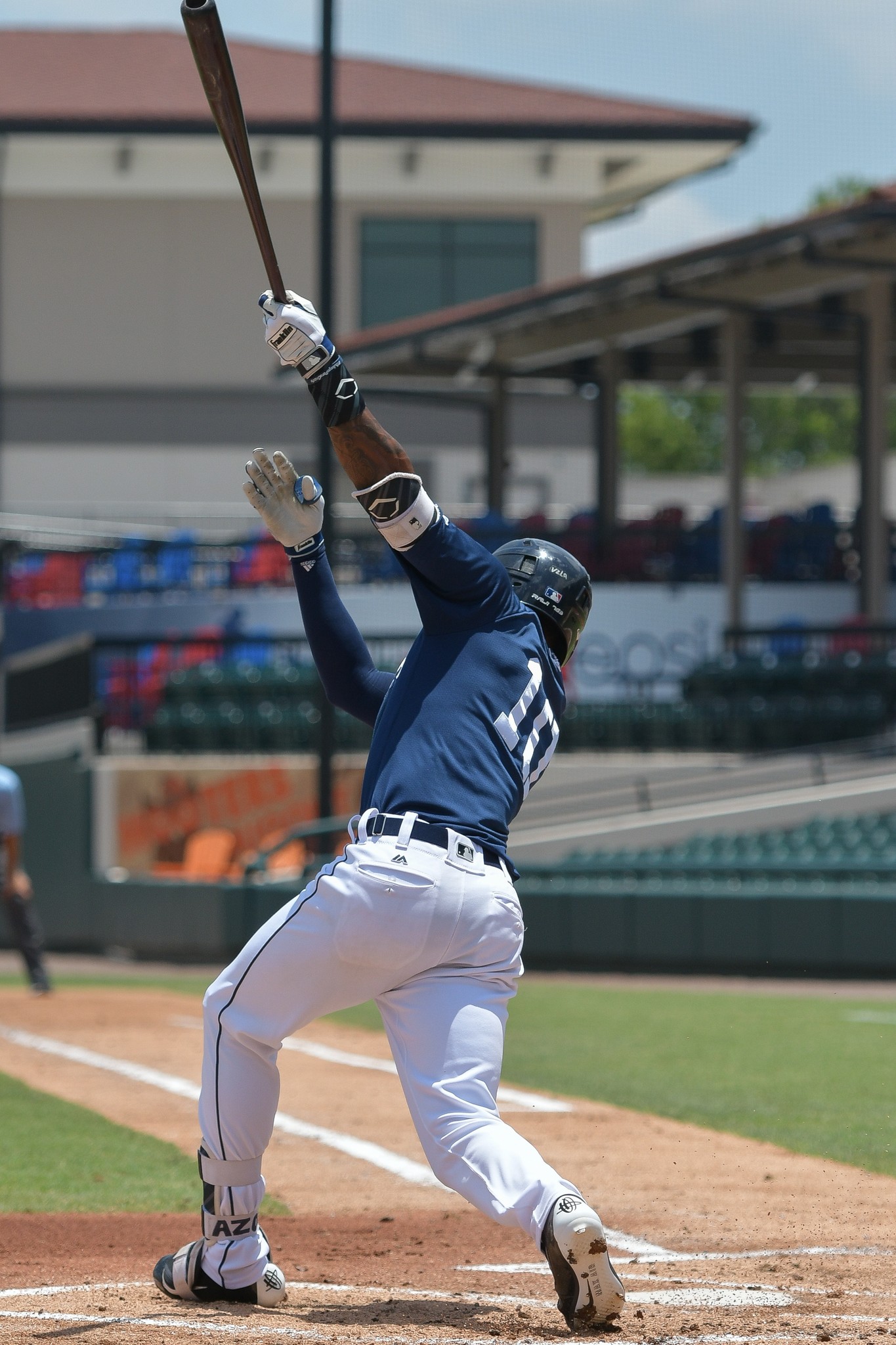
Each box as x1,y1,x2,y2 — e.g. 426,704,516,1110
153,296,624,1336
0,761,54,991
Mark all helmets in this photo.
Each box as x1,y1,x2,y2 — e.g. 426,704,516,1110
492,537,593,666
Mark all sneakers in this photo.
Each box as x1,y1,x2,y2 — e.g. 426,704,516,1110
540,1198,626,1329
154,1238,285,1306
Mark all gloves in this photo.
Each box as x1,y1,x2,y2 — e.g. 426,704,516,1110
242,447,325,559
255,288,338,382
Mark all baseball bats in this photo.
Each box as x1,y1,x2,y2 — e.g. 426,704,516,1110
181,0,294,306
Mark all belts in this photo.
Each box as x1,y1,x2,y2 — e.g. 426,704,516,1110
368,815,506,867
